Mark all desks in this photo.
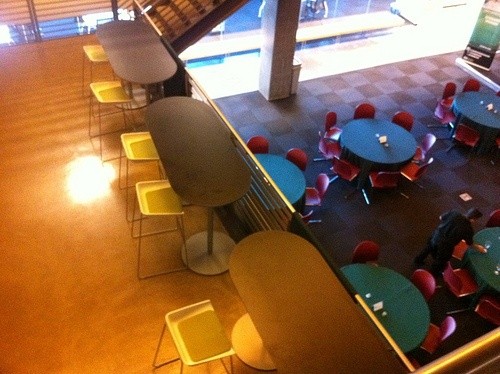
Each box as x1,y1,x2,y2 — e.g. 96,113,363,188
460,227,500,309
228,228,414,374
254,154,306,216
144,96,253,276
450,91,500,154
338,119,418,172
336,263,431,355
97,19,178,111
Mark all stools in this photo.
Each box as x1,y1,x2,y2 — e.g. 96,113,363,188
117,130,164,225
89,80,138,165
152,298,238,374
82,45,124,118
130,178,191,280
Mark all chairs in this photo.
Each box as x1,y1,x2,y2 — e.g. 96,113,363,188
247,77,500,369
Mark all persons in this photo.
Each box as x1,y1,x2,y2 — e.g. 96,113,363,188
413,207,486,276
